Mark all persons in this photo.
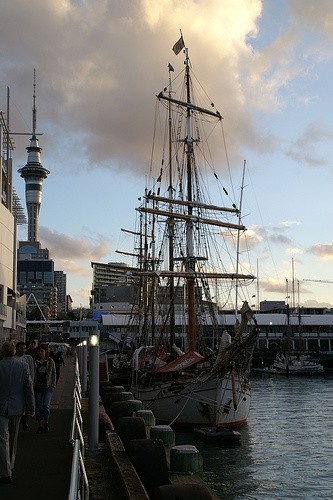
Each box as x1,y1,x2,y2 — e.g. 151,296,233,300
0,341,34,483
26,341,37,361
15,342,34,431
49,345,65,386
35,345,56,433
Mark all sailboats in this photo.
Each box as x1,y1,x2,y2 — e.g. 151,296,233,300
266,257,326,375
111,30,262,441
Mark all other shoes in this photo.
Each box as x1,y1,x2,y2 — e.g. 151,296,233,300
44,423,49,432
34,427,42,434
22,422,28,432
